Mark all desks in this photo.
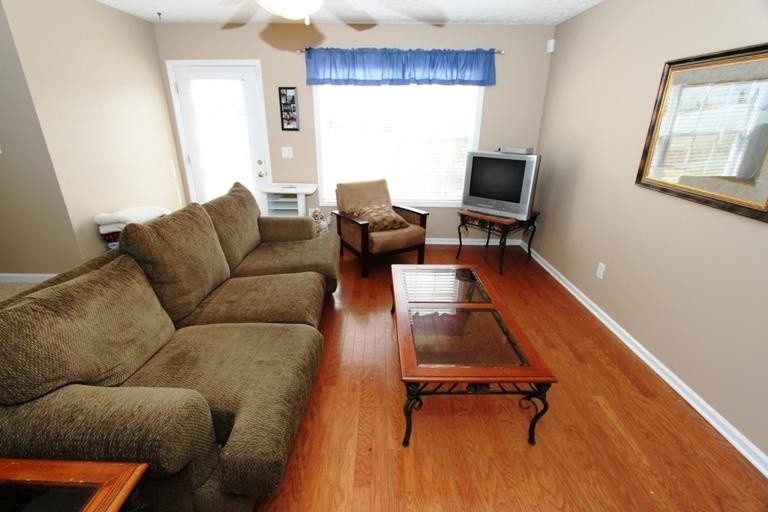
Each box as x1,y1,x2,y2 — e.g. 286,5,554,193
1,458,151,512
457,211,541,275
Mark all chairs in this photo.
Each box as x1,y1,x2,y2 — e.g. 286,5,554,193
331,179,429,278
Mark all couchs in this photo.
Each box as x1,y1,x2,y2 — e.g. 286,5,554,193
0,181,342,511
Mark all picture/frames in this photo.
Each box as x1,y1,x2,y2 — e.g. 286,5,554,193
632,43,768,222
278,86,300,131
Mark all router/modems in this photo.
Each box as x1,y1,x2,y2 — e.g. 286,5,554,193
500,147,533,155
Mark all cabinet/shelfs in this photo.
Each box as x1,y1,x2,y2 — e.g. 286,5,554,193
260,183,319,216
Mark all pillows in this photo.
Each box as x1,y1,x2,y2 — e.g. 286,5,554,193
347,202,409,233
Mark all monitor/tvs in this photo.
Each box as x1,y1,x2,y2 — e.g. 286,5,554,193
461,150,541,222
